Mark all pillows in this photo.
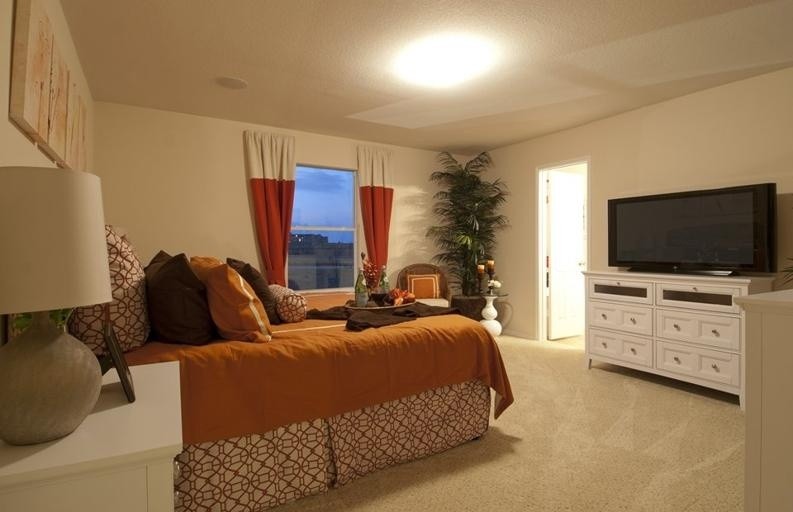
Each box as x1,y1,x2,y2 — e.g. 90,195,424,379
71,226,308,355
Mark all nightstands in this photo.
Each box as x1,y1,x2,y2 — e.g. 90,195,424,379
0,360,184,512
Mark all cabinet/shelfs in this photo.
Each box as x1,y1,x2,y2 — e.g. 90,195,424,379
580,266,776,410
732,289,793,512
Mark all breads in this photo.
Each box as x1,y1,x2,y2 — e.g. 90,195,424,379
385,287,415,306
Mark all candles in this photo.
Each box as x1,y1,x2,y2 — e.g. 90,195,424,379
477,264,485,274
487,260,494,271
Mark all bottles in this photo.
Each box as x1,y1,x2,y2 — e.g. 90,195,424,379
479,294,502,337
380,264,391,293
352,267,368,307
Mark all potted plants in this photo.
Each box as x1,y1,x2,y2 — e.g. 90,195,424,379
425,150,511,318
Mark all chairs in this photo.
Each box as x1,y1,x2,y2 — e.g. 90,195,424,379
398,263,450,307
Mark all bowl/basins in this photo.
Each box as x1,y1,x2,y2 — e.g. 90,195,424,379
344,298,418,315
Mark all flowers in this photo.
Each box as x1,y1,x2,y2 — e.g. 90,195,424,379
488,279,502,288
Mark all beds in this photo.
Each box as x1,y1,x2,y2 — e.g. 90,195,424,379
121,290,514,512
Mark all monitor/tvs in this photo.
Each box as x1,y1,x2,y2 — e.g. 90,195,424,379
608,182,776,276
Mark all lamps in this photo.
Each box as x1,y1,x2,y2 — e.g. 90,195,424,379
0,167,113,445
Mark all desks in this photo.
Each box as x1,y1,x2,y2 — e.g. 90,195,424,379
476,292,509,336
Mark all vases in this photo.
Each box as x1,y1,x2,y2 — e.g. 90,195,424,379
488,287,495,293
